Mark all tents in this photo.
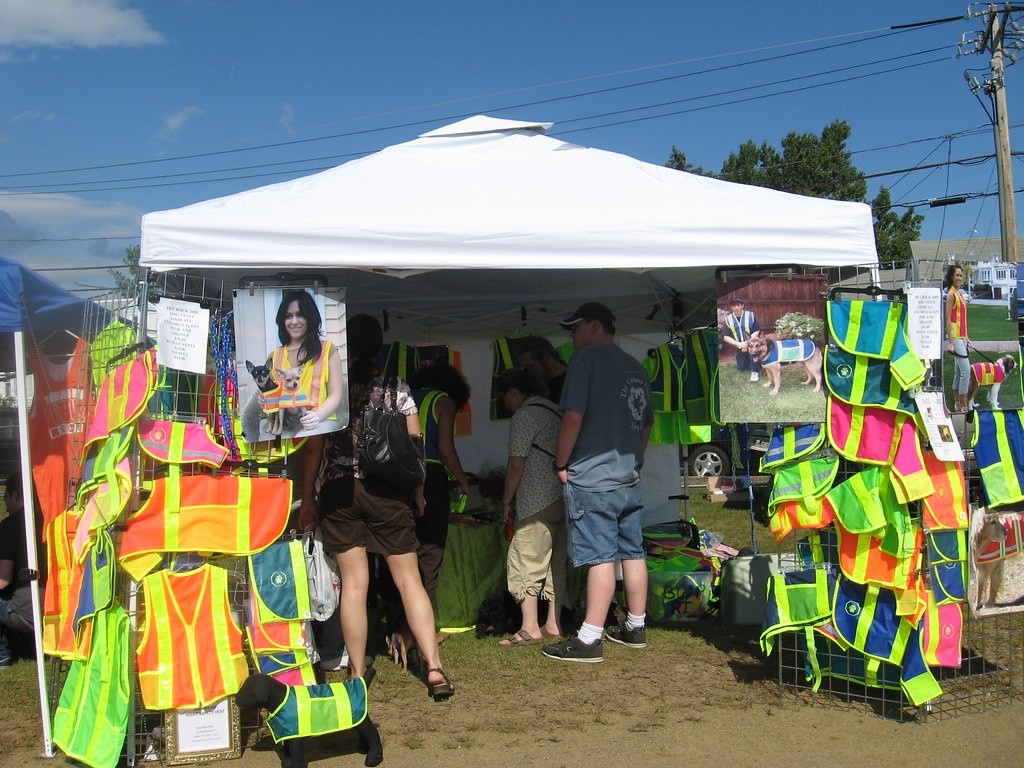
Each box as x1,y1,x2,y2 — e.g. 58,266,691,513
126,113,883,768
0,254,158,759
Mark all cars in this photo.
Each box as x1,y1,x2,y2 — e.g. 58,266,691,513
687,421,775,479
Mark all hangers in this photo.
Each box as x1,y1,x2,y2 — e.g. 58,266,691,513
489,318,532,348
142,391,207,425
382,323,450,348
943,229,999,266
280,529,315,543
66,478,85,533
208,440,291,483
134,552,245,590
828,273,903,310
242,269,322,297
26,321,93,358
647,322,686,361
776,543,832,577
106,335,146,370
780,447,839,465
721,265,797,282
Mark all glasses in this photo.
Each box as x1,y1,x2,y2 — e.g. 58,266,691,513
570,320,586,333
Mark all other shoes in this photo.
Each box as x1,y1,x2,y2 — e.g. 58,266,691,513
0,654,12,666
363,626,385,640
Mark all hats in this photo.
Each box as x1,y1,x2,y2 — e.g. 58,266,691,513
559,301,616,330
499,367,526,397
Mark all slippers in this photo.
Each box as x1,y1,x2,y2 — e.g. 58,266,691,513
496,629,544,645
538,625,561,639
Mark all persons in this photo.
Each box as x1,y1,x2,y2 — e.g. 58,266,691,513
721,296,760,382
0,472,46,668
298,314,455,698
409,364,471,598
258,290,343,442
319,553,374,671
496,369,565,646
542,302,654,663
945,266,972,413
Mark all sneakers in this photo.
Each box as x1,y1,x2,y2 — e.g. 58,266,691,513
606,622,646,648
542,634,603,662
318,649,373,670
749,371,759,382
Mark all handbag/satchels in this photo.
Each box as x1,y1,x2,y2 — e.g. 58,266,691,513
356,376,426,490
297,524,342,623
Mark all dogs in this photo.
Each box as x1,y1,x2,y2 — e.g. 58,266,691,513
245,358,307,436
746,329,824,395
234,666,385,768
970,506,1024,611
966,354,1018,410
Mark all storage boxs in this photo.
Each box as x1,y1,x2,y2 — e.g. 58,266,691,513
646,569,714,625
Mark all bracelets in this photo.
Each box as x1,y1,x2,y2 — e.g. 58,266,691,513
504,504,510,506
552,460,568,471
301,502,314,508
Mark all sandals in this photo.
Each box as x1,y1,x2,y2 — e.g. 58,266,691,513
426,669,455,696
961,403,968,413
954,400,961,412
